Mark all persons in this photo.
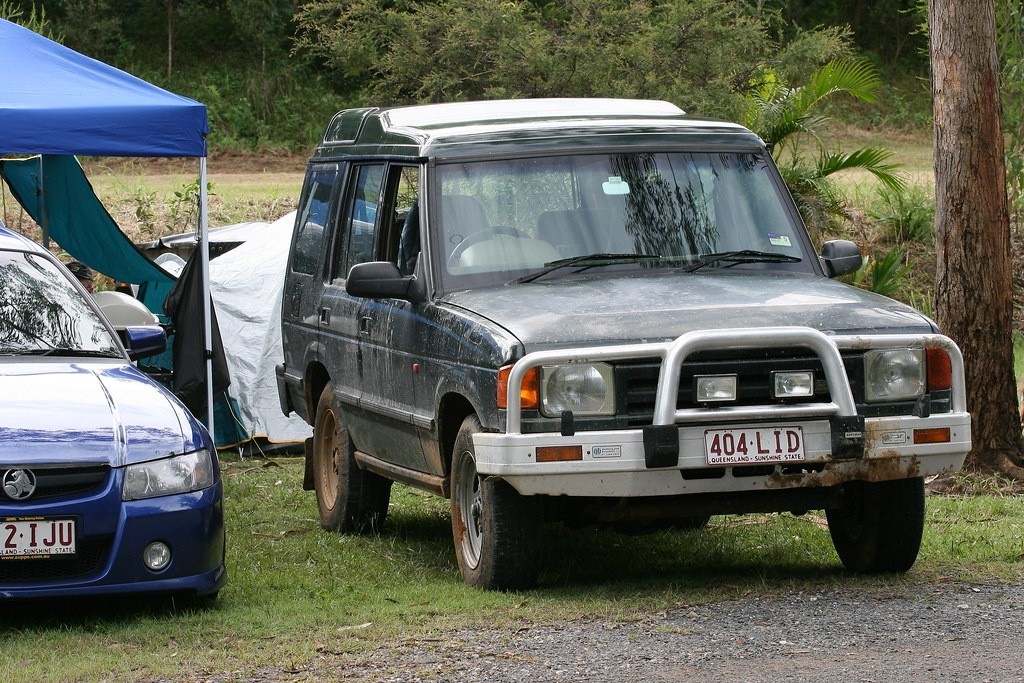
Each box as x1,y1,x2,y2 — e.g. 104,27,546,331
64,262,94,293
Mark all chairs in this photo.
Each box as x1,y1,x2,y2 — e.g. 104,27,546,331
529,207,620,261
396,196,491,275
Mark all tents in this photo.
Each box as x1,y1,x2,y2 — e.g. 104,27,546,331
0,16,215,447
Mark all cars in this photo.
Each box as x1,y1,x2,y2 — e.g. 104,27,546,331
0,226,225,609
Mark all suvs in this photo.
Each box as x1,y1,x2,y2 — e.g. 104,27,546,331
275,98,972,592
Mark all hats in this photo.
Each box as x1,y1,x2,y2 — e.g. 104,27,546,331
65,262,93,281
